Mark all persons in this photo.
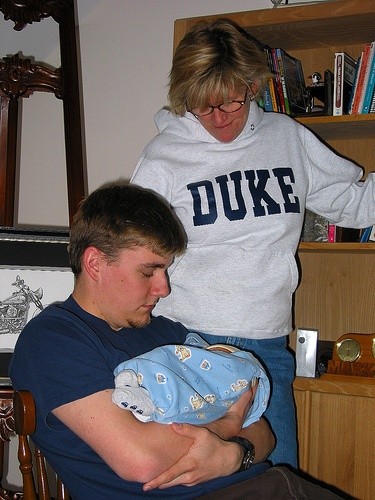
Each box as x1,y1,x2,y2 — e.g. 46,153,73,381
8,177,351,500
131,17,375,473
112,333,272,428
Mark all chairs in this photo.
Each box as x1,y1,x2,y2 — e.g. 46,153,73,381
15,390,71,500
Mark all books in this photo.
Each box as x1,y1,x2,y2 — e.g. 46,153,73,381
252,40,375,116
298,202,375,243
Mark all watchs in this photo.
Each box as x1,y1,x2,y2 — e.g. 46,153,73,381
228,435,258,474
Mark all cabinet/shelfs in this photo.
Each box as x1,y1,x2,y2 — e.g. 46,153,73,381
172,0,375,499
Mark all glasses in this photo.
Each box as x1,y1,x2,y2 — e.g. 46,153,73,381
186,86,248,116
